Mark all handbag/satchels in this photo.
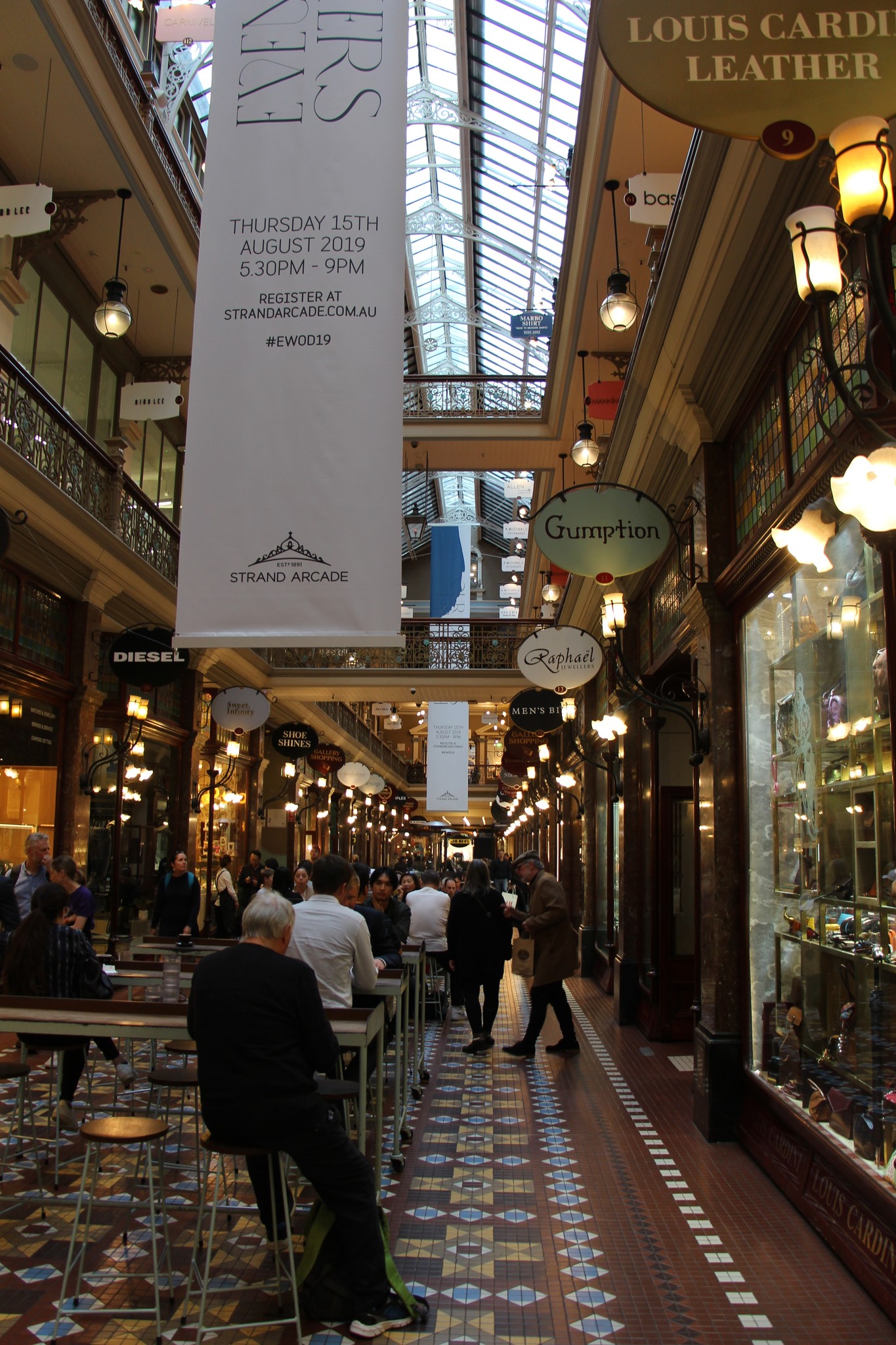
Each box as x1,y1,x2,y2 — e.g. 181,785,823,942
501,886,518,909
511,931,535,976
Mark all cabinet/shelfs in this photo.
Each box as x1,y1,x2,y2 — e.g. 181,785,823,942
767,588,896,1163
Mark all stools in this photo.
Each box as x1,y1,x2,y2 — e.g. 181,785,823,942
0,989,428,1345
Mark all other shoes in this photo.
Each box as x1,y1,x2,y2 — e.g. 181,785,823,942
481,1034,495,1045
462,1040,488,1054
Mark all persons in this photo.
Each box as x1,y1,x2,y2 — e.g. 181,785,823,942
362,866,411,945
150,850,201,939
237,849,280,937
0,881,140,1132
272,865,304,904
264,856,279,872
499,849,580,1058
308,845,323,864
107,864,142,935
350,853,360,864
0,875,22,930
293,866,315,901
350,862,408,1034
3,832,52,928
388,851,529,905
294,860,314,889
185,888,416,1339
283,853,395,1101
404,869,468,1021
238,850,265,908
256,867,279,898
45,855,95,948
443,858,514,1052
341,869,403,1033
350,854,367,871
214,854,238,937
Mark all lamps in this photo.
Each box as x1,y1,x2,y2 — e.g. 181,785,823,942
570,350,598,467
79,569,707,842
597,180,641,333
93,189,133,339
771,116,896,573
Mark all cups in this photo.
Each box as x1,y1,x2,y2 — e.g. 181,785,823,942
146,948,183,1003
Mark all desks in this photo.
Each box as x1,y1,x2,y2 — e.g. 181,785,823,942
131,939,431,1097
106,969,414,1172
0,994,384,1197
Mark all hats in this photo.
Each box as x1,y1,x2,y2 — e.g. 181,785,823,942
510,850,540,868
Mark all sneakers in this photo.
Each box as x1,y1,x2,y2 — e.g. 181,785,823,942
52,1100,79,1132
451,1008,468,1020
350,1300,412,1337
502,1041,535,1056
45,1051,58,1067
266,1189,295,1251
116,1061,139,1087
545,1039,579,1053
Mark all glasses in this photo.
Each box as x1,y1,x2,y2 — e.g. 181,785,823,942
515,863,523,872
176,850,184,853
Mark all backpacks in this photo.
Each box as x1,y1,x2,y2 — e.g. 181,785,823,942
106,905,132,936
292,1199,420,1321
